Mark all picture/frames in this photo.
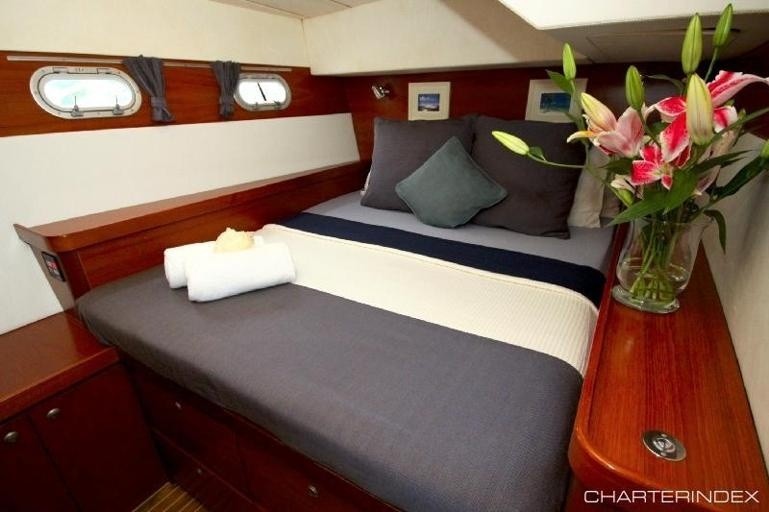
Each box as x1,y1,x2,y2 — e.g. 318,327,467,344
524,78,589,124
407,81,453,122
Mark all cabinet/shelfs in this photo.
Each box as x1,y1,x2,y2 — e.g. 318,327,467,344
0,309,171,512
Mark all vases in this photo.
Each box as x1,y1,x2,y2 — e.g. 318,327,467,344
612,216,713,315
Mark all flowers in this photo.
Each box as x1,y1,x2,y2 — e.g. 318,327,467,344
489,2,769,299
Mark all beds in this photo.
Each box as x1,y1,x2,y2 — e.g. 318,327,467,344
75,181,616,512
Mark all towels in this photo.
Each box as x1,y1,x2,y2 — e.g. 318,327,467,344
185,234,294,303
162,239,214,291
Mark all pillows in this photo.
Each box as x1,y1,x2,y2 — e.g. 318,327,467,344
470,114,584,241
566,144,610,229
359,116,474,220
393,135,508,229
